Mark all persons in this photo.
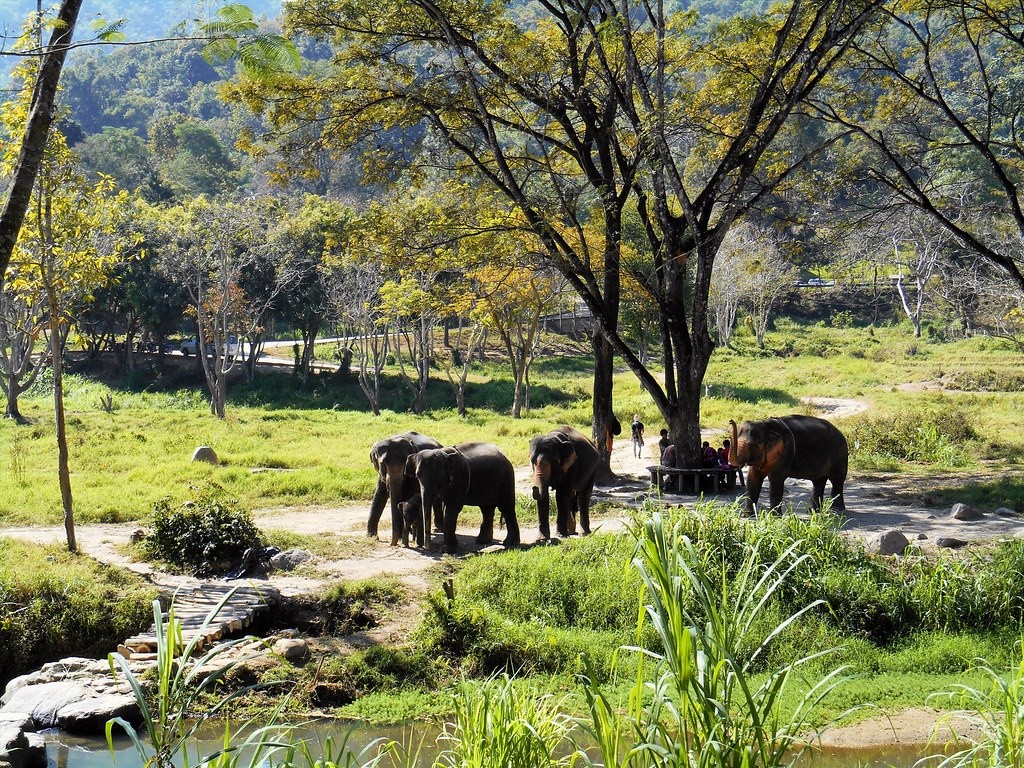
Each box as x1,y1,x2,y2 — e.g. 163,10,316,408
658,428,676,490
607,413,613,452
631,415,644,458
701,440,745,486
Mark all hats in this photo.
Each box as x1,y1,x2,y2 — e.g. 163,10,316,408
634,415,639,421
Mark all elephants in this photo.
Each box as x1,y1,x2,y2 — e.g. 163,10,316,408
528,425,602,544
728,415,848,520
366,431,520,556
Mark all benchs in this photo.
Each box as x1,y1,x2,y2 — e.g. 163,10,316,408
646,466,739,493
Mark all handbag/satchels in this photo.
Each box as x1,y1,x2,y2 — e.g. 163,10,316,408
722,465,730,470
